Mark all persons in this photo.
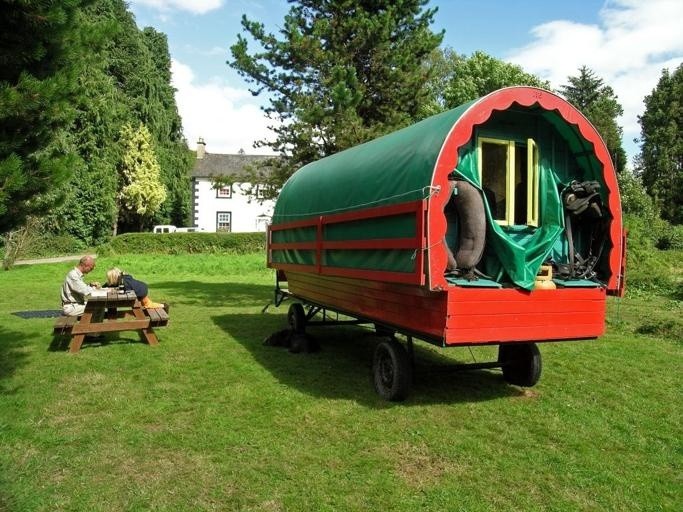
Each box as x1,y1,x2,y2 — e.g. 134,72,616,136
102,266,169,315
60,256,105,344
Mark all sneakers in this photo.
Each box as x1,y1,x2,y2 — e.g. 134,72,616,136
164,303,169,314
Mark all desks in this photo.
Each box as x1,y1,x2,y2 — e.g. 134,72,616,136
68,287,159,354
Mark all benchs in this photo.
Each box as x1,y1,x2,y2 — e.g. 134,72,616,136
53,312,78,334
144,307,171,329
